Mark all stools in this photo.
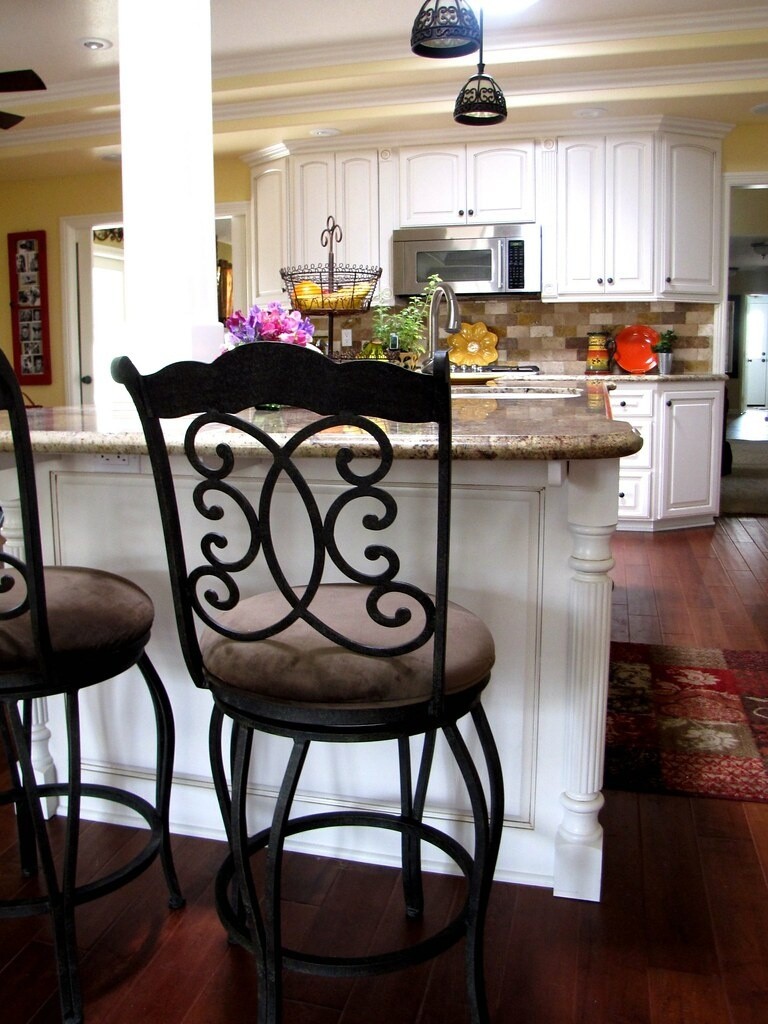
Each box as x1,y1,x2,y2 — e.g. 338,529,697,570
0,350,189,1024
111,341,504,1023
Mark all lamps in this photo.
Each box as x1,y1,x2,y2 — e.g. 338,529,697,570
749,242,768,259
452,8,508,126
409,0,482,59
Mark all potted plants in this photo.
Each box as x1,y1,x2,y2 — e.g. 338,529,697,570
371,273,444,370
651,330,678,375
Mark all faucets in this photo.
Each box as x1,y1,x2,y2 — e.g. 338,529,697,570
421,285,461,374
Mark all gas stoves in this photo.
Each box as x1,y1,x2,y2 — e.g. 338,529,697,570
448,364,541,372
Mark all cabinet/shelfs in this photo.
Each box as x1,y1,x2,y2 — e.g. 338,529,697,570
657,115,736,303
397,122,543,227
607,384,655,533
541,113,658,302
238,140,293,312
653,380,724,533
282,131,407,306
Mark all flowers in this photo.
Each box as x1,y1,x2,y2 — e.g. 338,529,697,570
222,300,315,348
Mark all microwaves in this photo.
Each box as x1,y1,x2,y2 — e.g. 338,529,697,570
393,224,540,300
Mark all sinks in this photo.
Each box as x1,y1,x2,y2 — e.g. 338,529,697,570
452,387,581,399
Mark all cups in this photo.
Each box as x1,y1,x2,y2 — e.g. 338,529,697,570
585,331,616,374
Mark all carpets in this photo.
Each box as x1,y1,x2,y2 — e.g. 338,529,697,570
602,637,768,803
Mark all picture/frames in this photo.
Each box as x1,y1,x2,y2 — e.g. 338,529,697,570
7,230,52,387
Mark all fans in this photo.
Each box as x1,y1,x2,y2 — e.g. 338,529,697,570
0,68,47,130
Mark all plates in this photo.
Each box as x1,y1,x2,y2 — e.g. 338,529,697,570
614,325,661,373
449,369,496,385
